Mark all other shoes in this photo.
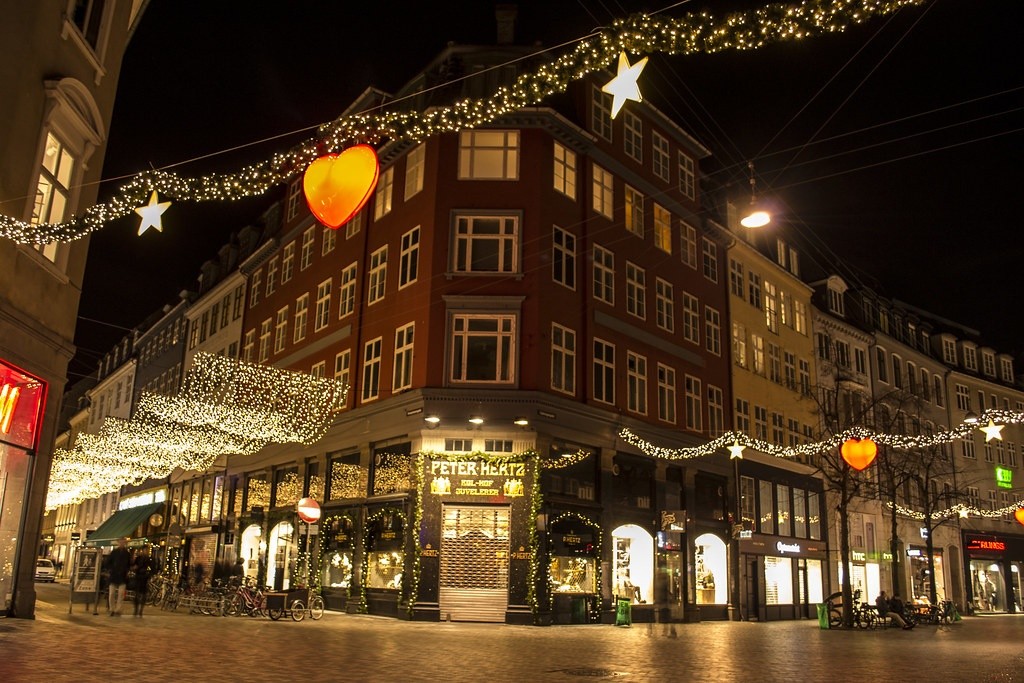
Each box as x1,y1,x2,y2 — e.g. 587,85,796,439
111,612,115,616
139,613,142,616
116,613,121,616
133,612,138,616
903,623,916,629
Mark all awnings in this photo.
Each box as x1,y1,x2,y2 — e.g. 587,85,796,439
84,502,162,542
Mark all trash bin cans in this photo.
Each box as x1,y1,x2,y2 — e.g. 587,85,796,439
551,592,587,625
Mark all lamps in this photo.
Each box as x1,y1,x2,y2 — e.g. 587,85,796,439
467,414,484,431
425,415,440,430
513,416,530,432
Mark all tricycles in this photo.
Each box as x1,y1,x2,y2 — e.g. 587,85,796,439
263,576,325,622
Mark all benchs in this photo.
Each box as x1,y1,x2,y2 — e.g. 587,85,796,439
913,604,934,625
863,605,893,630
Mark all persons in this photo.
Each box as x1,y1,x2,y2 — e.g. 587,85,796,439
990,591,997,610
133,546,157,618
102,537,132,618
211,557,244,584
876,591,915,630
624,569,646,603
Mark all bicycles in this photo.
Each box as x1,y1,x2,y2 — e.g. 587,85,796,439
128,570,267,617
827,600,879,631
908,599,956,625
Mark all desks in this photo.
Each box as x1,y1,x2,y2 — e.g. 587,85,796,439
696,588,715,604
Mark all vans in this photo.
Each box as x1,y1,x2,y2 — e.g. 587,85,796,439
35,559,56,583
